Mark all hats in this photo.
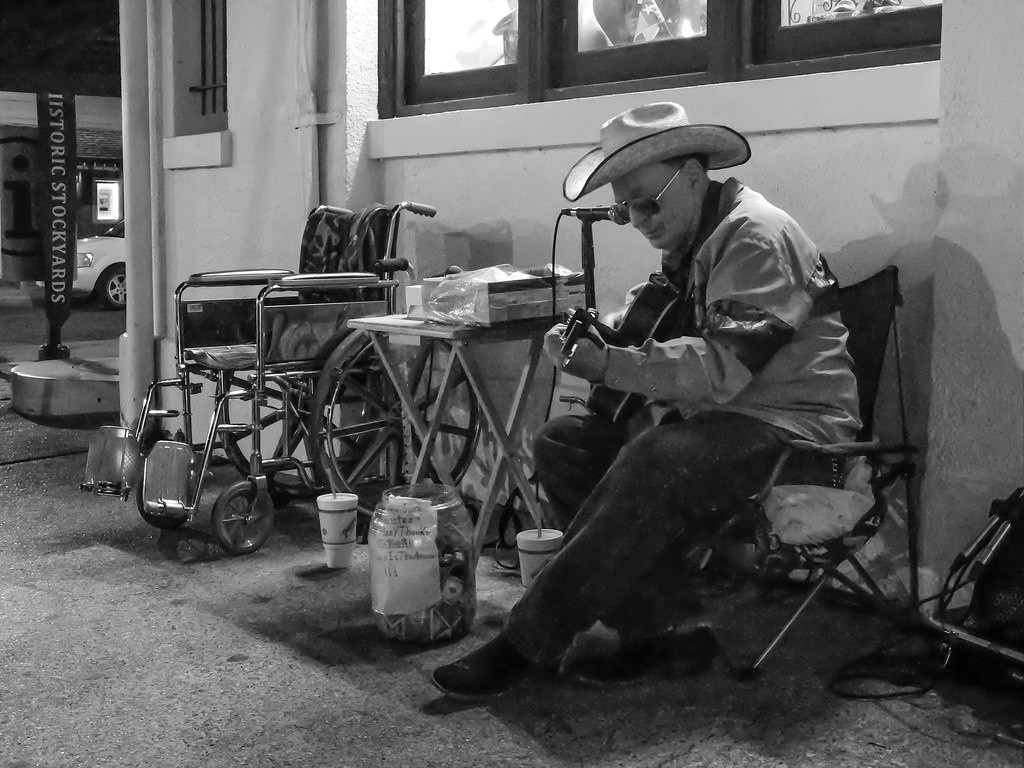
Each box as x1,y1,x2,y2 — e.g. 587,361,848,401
564,103,752,202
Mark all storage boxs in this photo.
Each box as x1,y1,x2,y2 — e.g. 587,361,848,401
405,268,587,328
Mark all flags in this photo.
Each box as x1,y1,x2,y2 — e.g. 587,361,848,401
625,0,670,42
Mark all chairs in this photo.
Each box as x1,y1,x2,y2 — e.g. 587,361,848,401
677,264,920,679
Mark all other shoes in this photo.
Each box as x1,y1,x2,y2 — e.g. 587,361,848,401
433,636,560,701
573,629,725,685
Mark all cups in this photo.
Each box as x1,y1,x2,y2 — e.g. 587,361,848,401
316,493,358,569
516,529,563,587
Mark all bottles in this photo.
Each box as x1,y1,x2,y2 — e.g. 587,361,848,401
369,483,476,643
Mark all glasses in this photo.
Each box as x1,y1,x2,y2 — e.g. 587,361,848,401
609,166,682,225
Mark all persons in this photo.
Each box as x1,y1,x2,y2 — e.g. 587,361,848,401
430,102,862,702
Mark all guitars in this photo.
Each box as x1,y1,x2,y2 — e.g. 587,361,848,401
556,280,684,426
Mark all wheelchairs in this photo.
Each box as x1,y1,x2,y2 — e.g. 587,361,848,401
69,202,480,555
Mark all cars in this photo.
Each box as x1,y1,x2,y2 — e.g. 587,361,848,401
69,216,128,310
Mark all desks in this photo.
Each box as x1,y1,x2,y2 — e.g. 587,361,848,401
346,313,569,569
